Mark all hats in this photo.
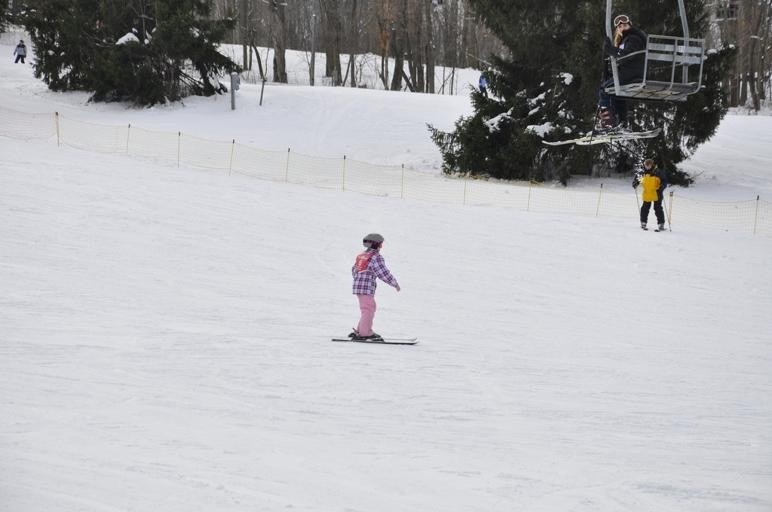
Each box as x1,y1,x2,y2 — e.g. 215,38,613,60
362,233,385,249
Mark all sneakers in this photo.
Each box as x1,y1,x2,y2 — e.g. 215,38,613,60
641,222,646,228
357,333,381,340
657,223,665,230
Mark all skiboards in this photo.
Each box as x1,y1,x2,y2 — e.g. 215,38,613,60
640,224,666,232
330,335,419,344
541,129,660,146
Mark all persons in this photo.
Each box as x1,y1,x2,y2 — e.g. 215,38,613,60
349,233,401,339
592,13,647,129
479,74,487,98
13,40,28,64
632,159,669,230
